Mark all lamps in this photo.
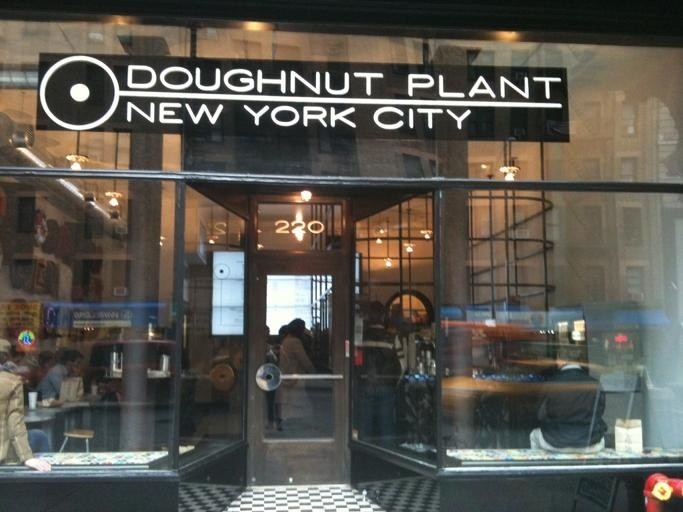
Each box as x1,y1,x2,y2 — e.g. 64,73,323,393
499,164,522,182
65,130,123,206
375,228,434,269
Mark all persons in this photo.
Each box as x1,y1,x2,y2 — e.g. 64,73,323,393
0,336,85,477
264,318,331,437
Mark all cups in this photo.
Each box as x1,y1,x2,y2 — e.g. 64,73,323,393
28,392,37,410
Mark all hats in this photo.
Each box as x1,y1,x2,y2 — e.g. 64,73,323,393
0,339,13,354
